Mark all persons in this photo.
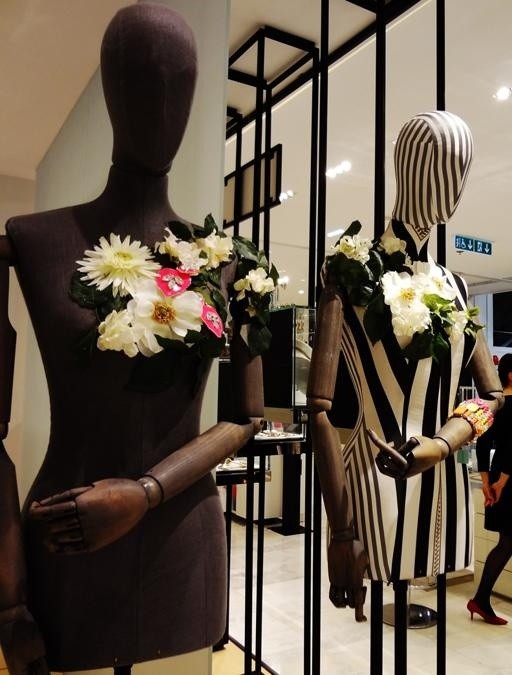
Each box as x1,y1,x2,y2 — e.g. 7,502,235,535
304,107,507,627
462,352,511,629
1,1,275,674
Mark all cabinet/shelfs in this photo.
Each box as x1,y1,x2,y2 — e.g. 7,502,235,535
222,0,447,675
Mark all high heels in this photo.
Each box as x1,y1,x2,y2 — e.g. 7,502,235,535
467,598,508,624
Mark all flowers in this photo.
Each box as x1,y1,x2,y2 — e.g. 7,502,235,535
327,235,486,359
69,213,279,358
